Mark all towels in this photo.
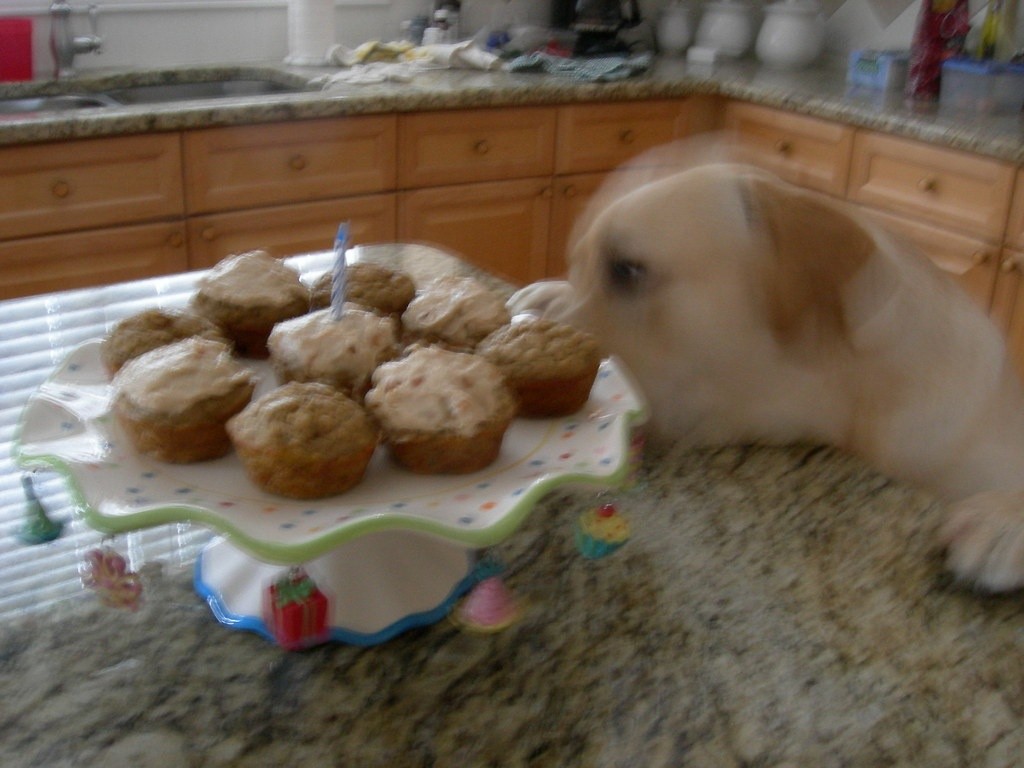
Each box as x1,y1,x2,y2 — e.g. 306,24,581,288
307,38,656,95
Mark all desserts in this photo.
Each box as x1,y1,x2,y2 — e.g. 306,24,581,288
102,251,600,500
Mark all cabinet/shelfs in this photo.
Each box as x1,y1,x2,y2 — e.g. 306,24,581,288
399,94,722,291
723,94,1023,371
1,111,399,302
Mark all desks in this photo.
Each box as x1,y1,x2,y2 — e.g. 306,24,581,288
0,241,1023,768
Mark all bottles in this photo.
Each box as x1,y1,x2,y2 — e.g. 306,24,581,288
656,3,693,57
756,3,824,72
421,27,441,48
432,9,453,46
696,2,756,59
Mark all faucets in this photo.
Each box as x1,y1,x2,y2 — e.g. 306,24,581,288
45,0,103,83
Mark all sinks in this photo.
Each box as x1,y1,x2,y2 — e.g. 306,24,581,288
83,66,334,112
0,67,110,121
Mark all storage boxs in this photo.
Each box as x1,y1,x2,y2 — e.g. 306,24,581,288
846,46,910,91
940,56,1024,121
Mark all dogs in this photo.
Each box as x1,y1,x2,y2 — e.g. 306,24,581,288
503,161,1024,600
